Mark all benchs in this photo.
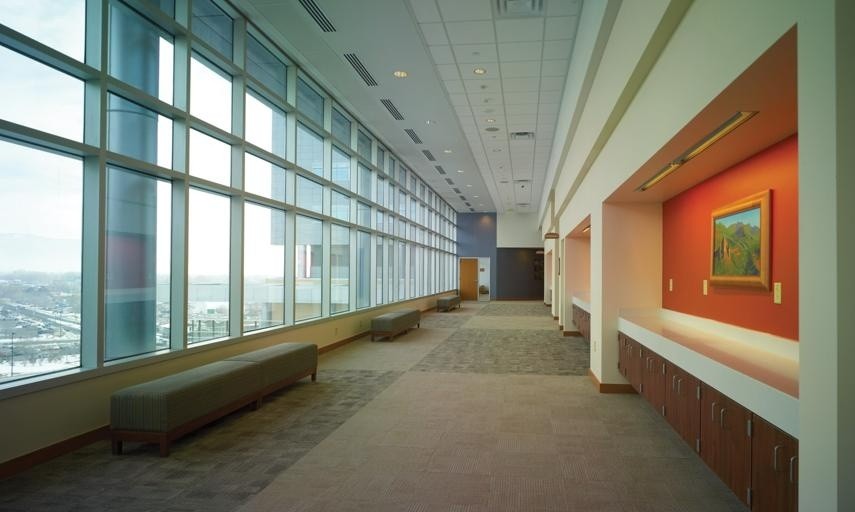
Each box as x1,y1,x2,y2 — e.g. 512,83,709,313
108,342,319,458
368,295,463,343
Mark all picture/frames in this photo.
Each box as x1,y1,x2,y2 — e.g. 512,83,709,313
708,187,773,289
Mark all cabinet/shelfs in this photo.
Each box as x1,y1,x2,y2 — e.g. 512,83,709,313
617,331,799,511
